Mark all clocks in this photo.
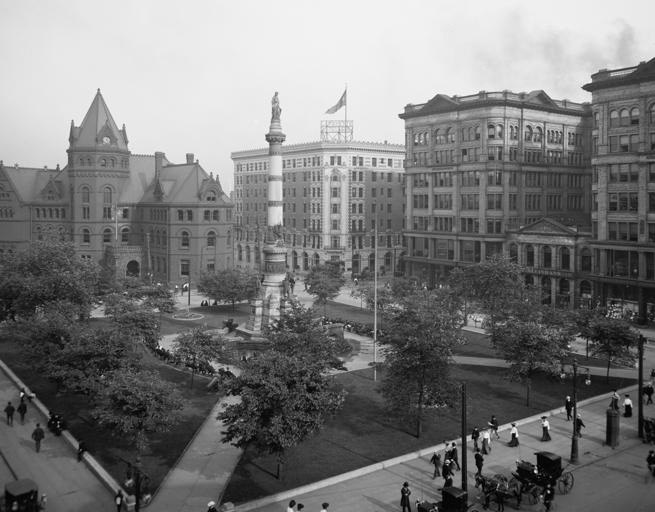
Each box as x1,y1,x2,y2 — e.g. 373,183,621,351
103,136,110,144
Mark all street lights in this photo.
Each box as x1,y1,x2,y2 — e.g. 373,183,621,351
560,359,591,464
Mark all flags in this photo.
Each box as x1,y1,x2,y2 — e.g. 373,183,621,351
326,91,347,116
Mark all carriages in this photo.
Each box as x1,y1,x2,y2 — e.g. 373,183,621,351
475,452,574,511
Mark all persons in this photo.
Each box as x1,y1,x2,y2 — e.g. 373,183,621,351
430,412,500,488
400,481,413,511
207,501,217,512
541,416,551,441
497,474,508,511
285,499,297,512
642,368,655,476
45,409,68,438
270,90,283,122
31,422,46,453
573,413,585,438
608,388,621,410
509,422,520,447
26,391,37,402
565,395,573,420
295,503,304,512
18,399,29,425
326,317,390,345
320,503,329,512
76,438,90,462
3,400,16,426
541,482,555,512
19,387,25,401
154,343,239,379
622,393,633,417
114,489,123,512
